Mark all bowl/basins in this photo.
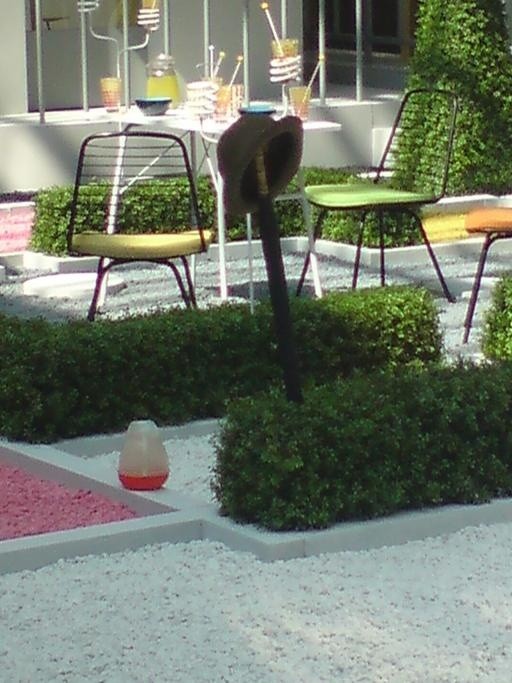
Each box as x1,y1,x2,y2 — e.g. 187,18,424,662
237,105,279,115
135,99,173,115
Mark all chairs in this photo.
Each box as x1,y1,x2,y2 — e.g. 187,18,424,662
296,89,459,301
67,132,214,322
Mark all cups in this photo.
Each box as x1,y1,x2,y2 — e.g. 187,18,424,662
101,77,120,112
271,38,298,83
290,85,310,122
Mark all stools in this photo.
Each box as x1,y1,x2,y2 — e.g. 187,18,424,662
461,209,512,343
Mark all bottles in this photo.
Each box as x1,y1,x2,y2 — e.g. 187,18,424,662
145,55,181,111
117,410,169,490
